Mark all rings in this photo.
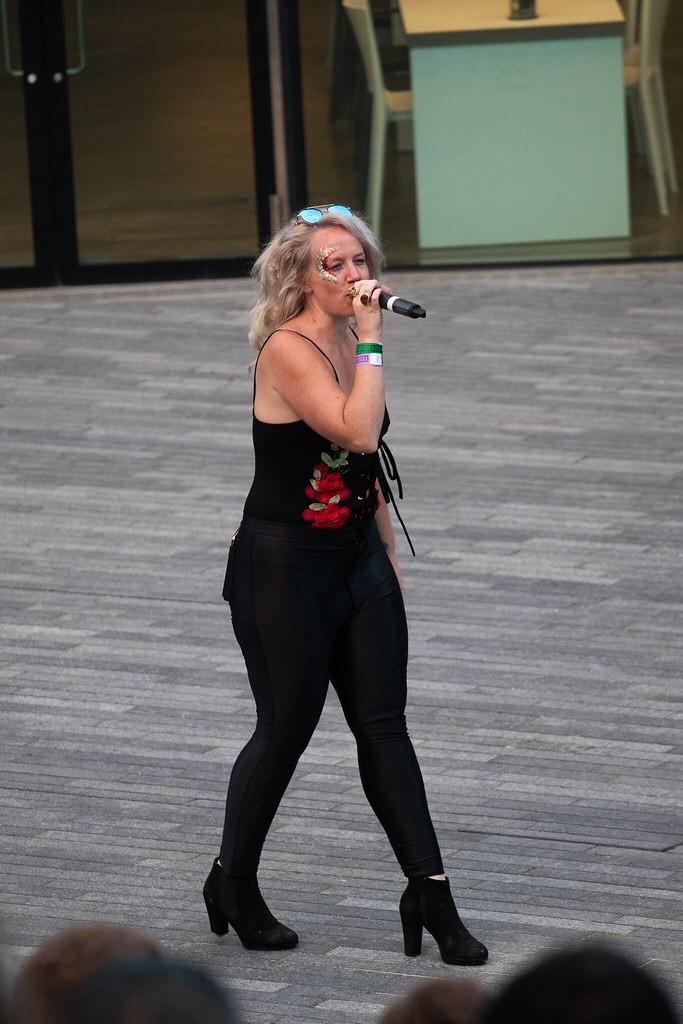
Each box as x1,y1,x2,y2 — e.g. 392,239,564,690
347,286,359,296
360,293,371,305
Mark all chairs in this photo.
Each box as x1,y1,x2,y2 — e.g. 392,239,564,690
623,0,679,217
342,0,413,245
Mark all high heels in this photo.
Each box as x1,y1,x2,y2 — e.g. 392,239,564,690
399,876,488,966
202,857,298,952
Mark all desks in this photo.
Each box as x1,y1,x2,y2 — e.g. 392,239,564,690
389,0,631,251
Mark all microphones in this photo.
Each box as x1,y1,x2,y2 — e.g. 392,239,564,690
370,288,427,320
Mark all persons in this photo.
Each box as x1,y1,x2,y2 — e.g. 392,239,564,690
201,207,492,966
382,977,496,1024
476,941,683,1024
10,929,240,1023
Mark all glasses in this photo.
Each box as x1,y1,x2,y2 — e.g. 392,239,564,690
292,204,353,226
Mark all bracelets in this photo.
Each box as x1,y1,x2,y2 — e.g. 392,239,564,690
354,342,384,367
